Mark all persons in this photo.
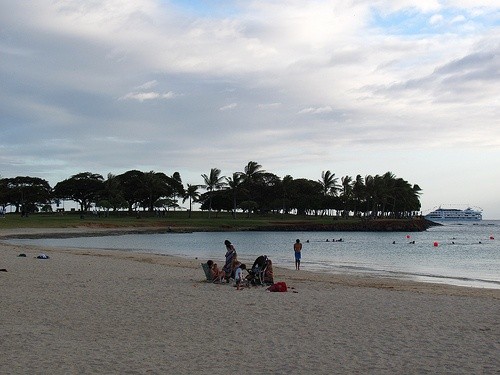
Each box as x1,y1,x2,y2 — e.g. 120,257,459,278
235,264,246,290
207,260,227,284
222,240,242,284
294,239,302,270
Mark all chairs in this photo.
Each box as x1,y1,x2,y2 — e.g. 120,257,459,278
201,263,221,284
246,255,269,284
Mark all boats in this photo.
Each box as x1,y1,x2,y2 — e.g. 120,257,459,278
425,205,484,221
